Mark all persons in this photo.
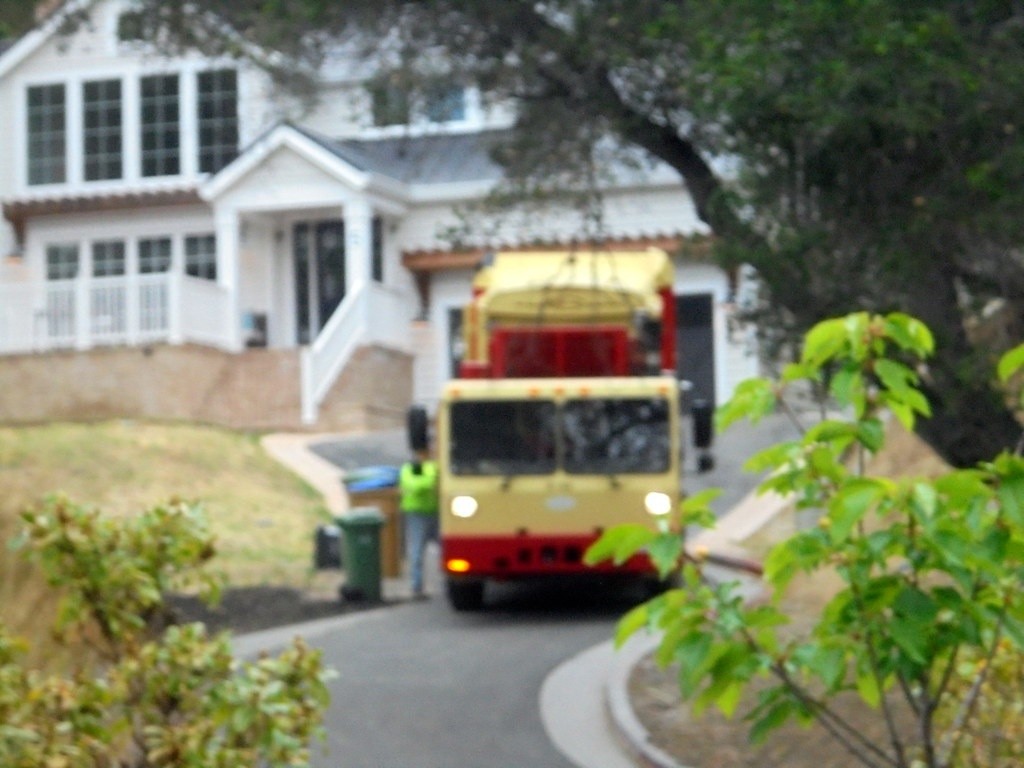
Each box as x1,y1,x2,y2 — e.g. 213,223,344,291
401,449,439,601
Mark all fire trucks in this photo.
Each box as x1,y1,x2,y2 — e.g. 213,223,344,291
437,246,682,611
